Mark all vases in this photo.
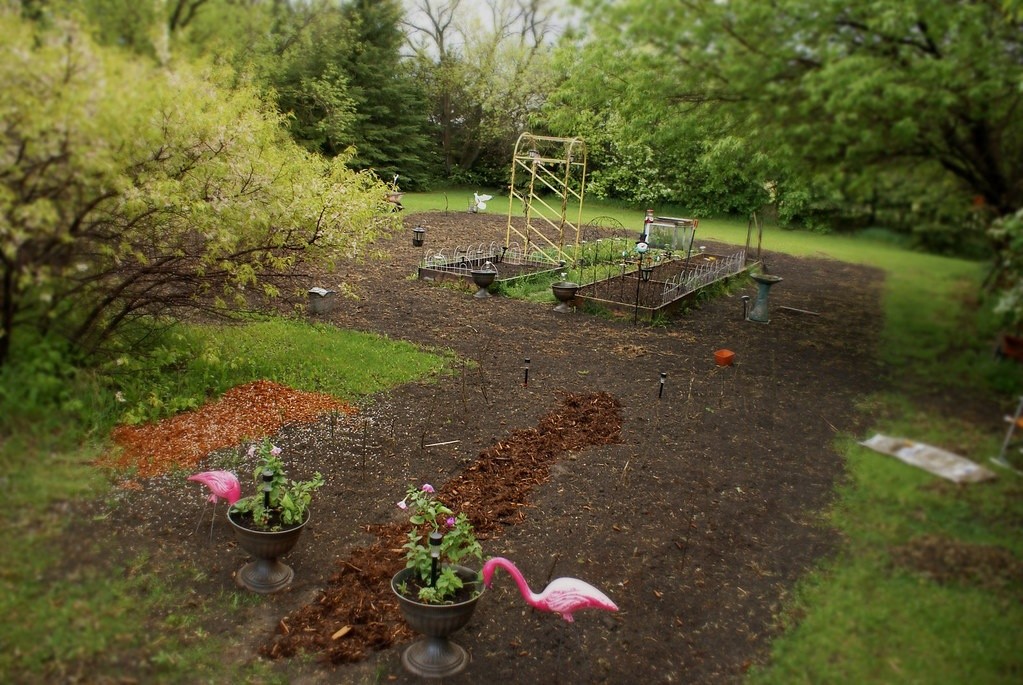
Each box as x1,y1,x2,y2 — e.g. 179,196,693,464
390,564,488,679
226,496,311,594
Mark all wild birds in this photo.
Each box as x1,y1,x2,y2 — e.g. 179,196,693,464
186,470,242,546
483,558,620,685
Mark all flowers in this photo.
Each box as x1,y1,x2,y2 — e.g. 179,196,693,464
230,431,326,531
395,482,507,604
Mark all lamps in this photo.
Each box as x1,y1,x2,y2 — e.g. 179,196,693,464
411,224,426,281
